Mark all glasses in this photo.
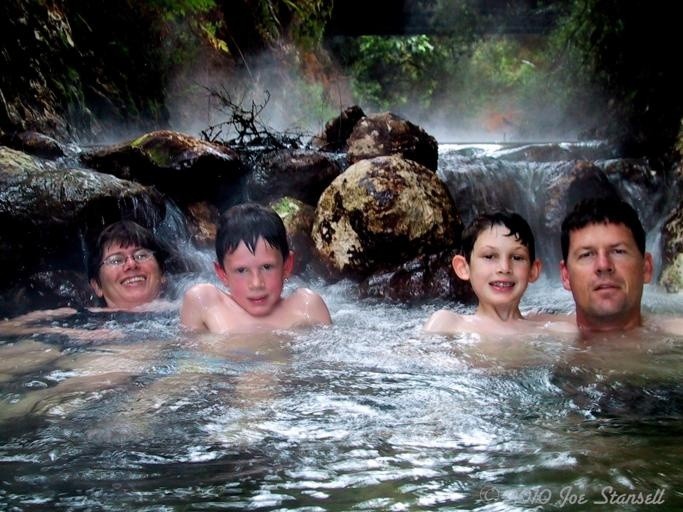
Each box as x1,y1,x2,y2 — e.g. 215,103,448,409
99,248,155,268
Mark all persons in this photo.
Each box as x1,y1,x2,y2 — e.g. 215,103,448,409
0,217,178,424
405,204,682,352
524,194,664,374
114,202,350,455
